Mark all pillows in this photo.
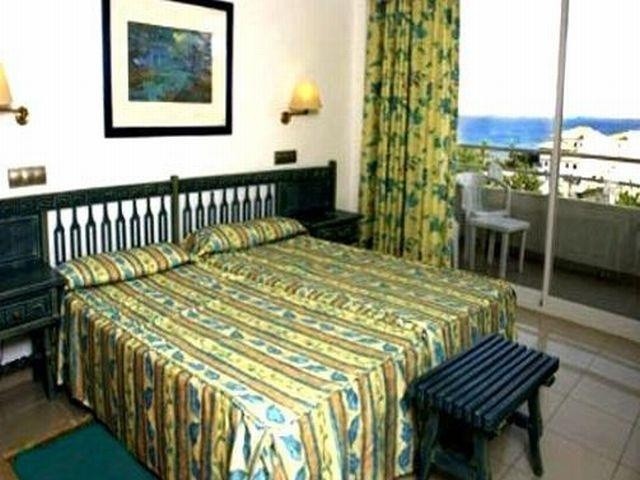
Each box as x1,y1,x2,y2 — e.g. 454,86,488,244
55,211,312,292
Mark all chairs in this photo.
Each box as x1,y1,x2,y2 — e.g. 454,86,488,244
453,171,532,284
42,169,521,480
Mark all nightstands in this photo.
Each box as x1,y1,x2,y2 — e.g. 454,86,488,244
295,207,364,244
0,253,67,401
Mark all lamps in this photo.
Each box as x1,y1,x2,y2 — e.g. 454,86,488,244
0,62,31,126
280,77,323,126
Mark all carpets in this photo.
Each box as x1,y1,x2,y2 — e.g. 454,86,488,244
3,415,162,480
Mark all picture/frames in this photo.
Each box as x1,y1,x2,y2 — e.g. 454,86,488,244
100,1,236,141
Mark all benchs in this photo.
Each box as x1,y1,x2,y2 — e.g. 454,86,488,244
406,330,561,480
